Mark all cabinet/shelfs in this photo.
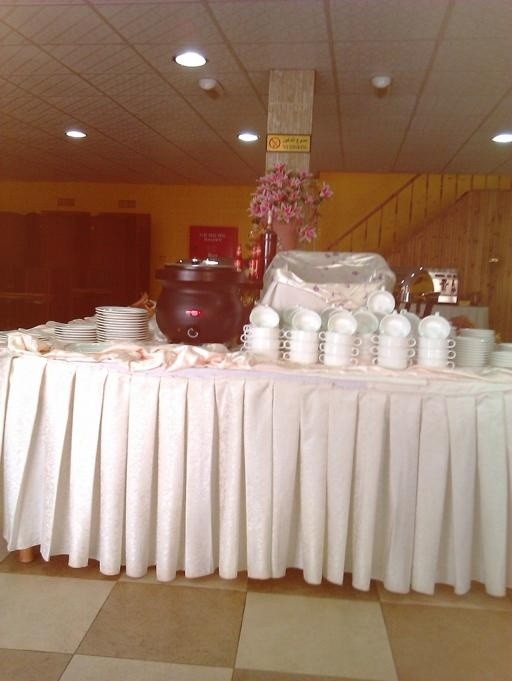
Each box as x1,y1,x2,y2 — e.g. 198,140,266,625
0,210,151,331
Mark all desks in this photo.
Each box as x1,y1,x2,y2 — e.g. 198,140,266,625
0,319,512,597
398,302,490,329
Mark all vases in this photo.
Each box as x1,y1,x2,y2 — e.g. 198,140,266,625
273,223,298,249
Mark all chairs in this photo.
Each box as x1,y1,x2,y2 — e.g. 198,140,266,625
405,295,435,319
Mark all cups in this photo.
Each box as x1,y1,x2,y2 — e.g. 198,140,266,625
240,286,456,371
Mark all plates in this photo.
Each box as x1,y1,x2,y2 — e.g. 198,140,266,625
0,305,150,345
456,328,512,369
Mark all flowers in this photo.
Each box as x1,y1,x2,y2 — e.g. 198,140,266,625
246,161,334,243
